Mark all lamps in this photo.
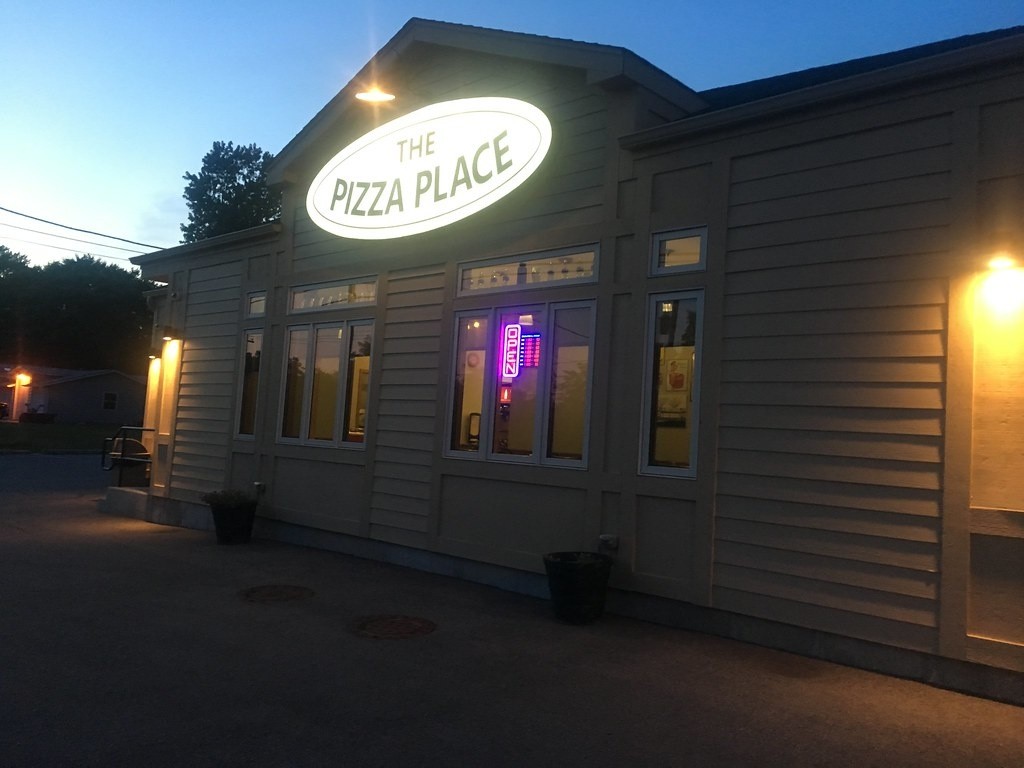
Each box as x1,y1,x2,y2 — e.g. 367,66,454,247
148,348,156,359
163,326,172,341
502,276,510,285
478,280,483,288
562,267,568,279
576,267,584,277
532,272,540,282
547,271,554,279
491,278,497,287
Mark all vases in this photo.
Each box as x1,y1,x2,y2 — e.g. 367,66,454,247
544,551,612,624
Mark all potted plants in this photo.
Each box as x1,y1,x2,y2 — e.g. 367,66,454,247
199,489,256,545
